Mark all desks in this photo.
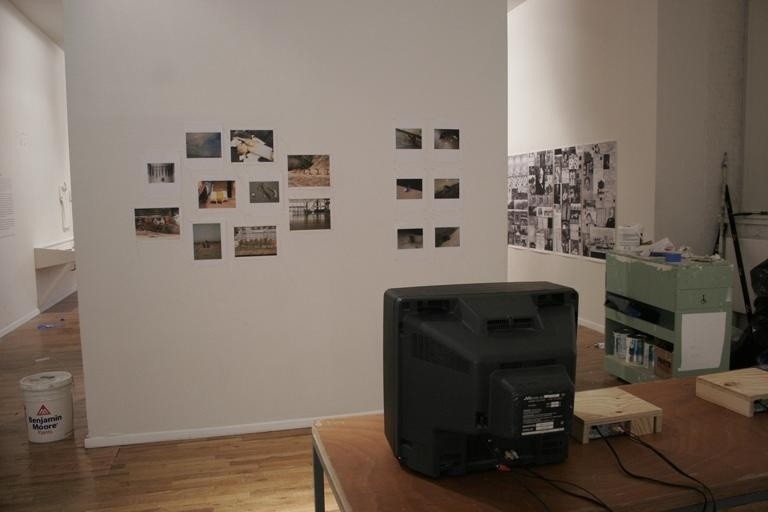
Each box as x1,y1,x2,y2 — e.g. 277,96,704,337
310,371,767,512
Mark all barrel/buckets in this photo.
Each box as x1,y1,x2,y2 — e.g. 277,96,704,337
618,225,641,248
20,357,73,443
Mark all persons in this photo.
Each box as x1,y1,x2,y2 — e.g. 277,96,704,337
508,144,616,259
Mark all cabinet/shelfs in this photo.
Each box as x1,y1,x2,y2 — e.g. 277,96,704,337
602,251,732,383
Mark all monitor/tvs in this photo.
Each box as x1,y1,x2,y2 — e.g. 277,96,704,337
383,281,579,479
